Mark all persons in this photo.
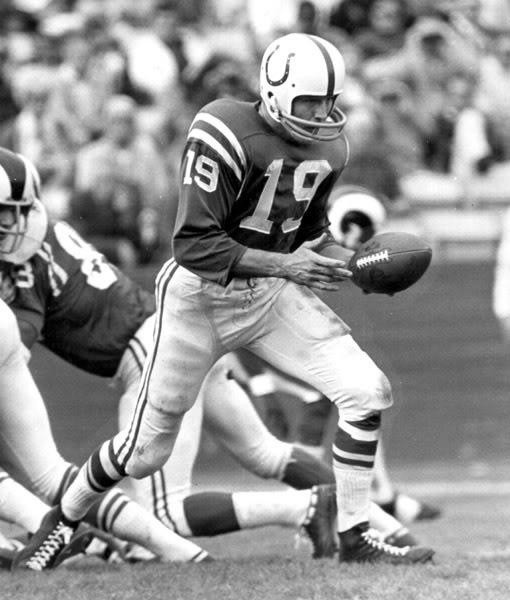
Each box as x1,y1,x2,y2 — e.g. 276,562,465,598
0,0,509,573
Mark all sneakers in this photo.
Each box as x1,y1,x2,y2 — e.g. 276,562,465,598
339,495,439,564
10,527,92,564
302,484,335,557
13,502,86,572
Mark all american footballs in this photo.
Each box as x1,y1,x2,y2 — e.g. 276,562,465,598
347,233,431,294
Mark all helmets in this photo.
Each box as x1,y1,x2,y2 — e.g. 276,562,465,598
0,146,36,254
259,34,347,144
0,200,47,266
